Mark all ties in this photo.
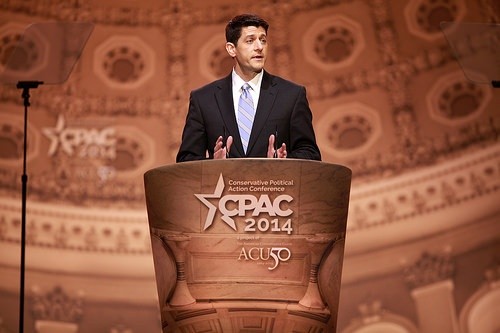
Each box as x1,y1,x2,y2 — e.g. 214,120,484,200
237,83,256,155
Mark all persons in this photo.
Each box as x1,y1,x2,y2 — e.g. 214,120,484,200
174,12,322,164
